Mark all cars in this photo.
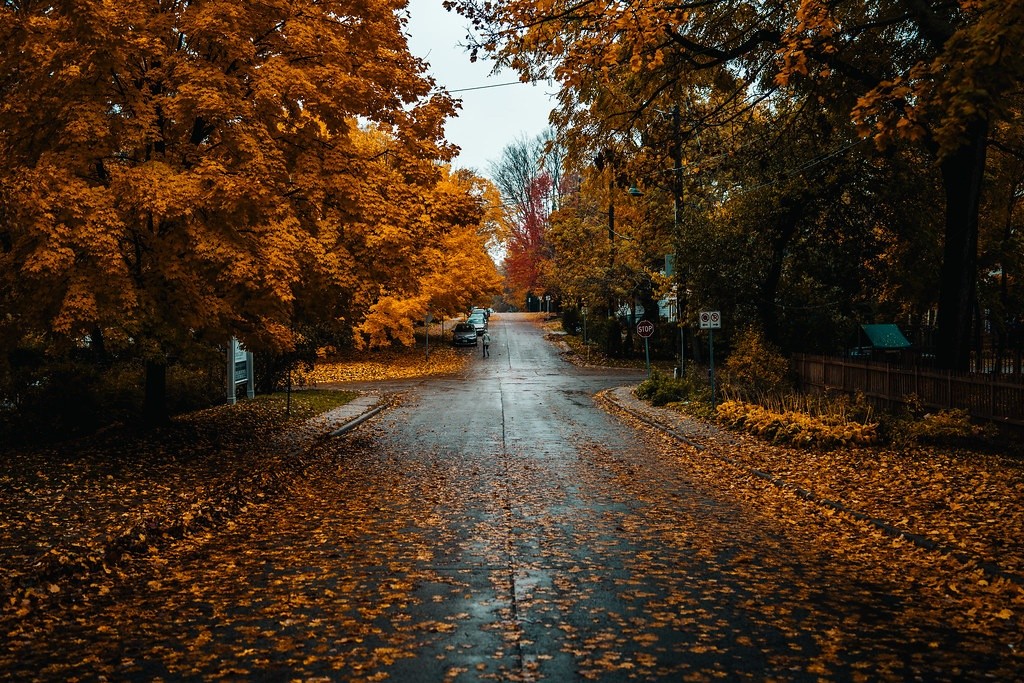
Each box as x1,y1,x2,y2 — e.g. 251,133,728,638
465,306,494,335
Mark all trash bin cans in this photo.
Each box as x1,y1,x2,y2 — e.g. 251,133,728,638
674,368,682,379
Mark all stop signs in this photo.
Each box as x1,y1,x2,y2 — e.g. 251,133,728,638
636,320,655,338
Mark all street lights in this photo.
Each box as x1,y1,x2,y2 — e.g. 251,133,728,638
629,170,684,321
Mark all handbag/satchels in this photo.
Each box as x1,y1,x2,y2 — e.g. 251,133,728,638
484,341,491,345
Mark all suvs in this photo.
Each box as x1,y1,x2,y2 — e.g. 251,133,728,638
449,323,479,347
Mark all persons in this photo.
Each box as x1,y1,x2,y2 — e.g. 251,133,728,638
482,329,490,357
